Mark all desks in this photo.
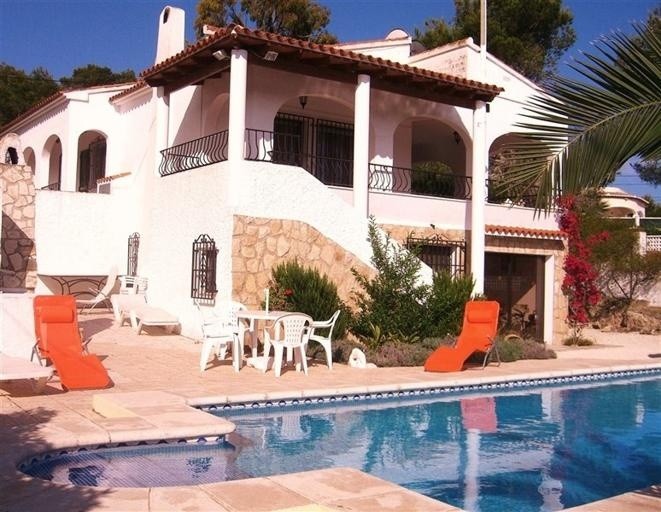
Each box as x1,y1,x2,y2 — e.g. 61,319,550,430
35,272,126,308
235,309,296,370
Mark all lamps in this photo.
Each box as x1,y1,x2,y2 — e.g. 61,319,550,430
211,49,229,61
299,95,306,109
453,131,460,144
263,51,278,63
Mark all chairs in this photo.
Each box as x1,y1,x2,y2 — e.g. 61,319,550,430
75,270,120,316
459,396,498,434
29,294,114,390
263,312,314,377
295,309,340,372
423,300,500,372
217,300,253,360
110,293,179,336
193,297,241,372
